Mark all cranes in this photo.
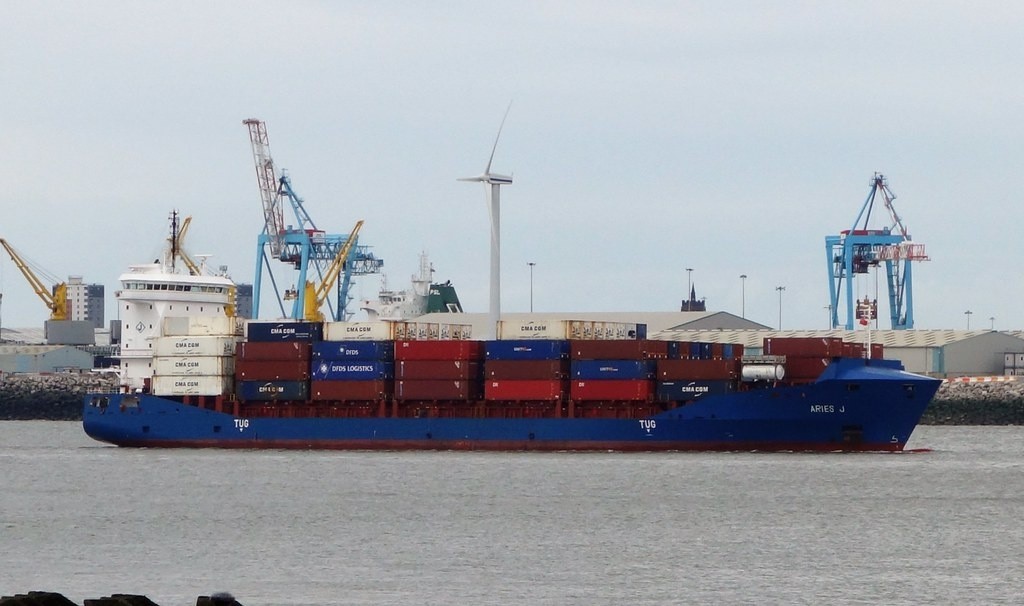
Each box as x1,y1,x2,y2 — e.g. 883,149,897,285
0,239,96,343
303,218,364,320
242,115,385,320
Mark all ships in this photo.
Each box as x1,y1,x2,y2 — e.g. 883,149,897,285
77,207,939,456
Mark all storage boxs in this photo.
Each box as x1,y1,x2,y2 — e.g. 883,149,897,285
149,314,884,420
